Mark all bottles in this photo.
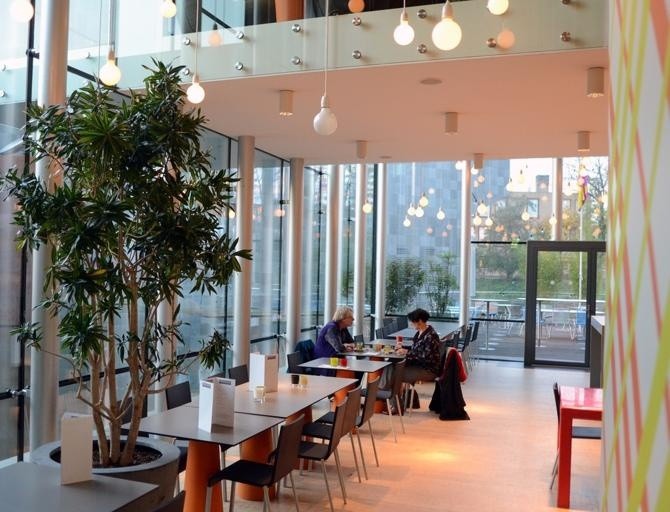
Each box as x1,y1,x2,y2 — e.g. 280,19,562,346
383,343,391,353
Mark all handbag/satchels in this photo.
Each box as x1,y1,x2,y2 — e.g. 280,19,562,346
400,388,422,410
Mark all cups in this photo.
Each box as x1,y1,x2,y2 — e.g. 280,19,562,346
396,336,403,347
353,341,363,350
394,344,402,352
330,358,348,366
300,375,308,389
252,384,266,404
372,342,382,352
291,374,300,387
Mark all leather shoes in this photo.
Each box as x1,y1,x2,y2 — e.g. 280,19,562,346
381,401,405,417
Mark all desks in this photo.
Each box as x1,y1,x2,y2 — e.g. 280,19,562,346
555,384,606,507
184,372,359,501
120,406,287,512
2,459,159,510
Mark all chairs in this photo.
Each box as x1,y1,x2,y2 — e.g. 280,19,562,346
453,304,592,343
547,381,604,486
206,413,307,511
110,364,252,496
314,374,381,481
298,384,364,483
285,319,481,446
264,396,348,511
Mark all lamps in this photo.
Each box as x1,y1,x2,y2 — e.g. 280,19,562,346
360,158,558,246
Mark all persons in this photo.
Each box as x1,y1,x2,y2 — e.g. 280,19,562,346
312,306,354,376
377,308,440,416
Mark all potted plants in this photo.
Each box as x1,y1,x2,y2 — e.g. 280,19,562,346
0,62,251,510
